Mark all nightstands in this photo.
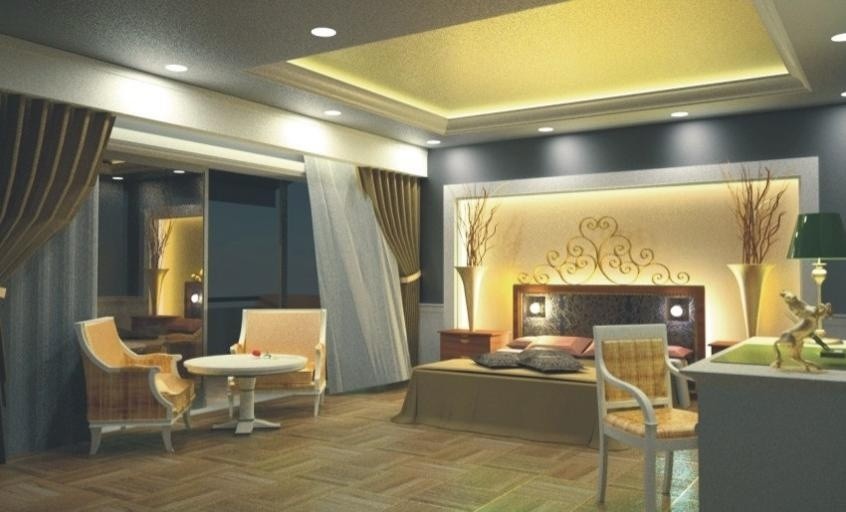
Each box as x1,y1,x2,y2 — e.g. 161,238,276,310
707,340,739,355
436,329,508,362
129,315,173,331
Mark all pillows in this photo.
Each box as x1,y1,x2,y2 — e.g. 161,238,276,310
167,316,204,336
513,349,587,374
117,325,161,342
525,334,592,357
471,351,523,370
579,338,693,361
507,335,535,349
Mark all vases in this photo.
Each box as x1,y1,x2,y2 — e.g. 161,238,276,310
454,266,482,332
146,267,167,315
726,260,772,339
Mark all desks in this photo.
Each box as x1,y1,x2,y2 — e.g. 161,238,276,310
182,353,309,436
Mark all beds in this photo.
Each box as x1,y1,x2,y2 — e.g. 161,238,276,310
390,283,707,452
119,280,205,352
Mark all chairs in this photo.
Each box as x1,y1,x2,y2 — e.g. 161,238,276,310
142,320,203,394
591,318,698,512
75,313,195,458
225,306,328,418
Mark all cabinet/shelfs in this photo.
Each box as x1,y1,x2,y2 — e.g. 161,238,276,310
679,334,844,512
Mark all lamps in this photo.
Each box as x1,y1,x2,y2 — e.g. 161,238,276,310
783,212,844,340
665,297,690,323
524,296,546,319
